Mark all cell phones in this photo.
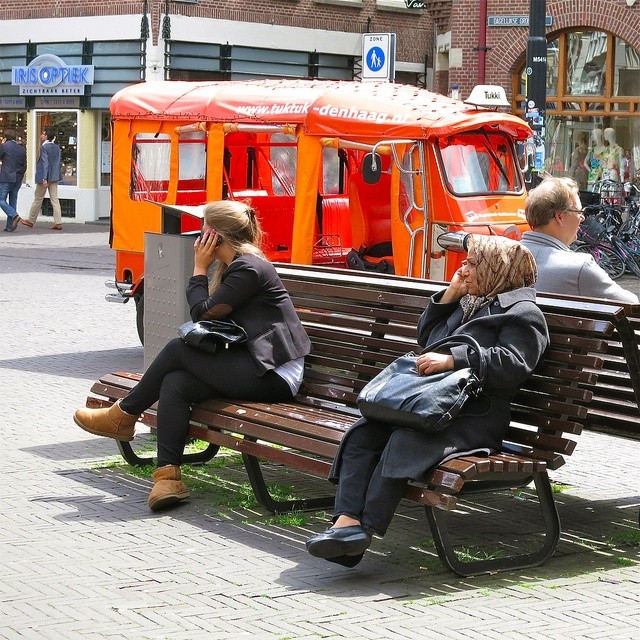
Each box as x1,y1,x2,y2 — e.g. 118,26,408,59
199,233,224,248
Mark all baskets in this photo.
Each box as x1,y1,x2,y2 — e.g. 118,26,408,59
579,213,606,246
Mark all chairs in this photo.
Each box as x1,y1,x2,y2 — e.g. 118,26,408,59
349,171,409,264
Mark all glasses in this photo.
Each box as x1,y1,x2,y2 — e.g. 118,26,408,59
555,207,587,217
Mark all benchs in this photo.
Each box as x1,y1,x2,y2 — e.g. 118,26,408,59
270,261,640,441
85,268,623,576
235,195,350,263
132,189,207,205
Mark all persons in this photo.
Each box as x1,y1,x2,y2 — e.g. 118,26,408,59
305,233,552,568
72,201,312,510
518,176,639,305
20,125,62,230
567,129,628,204
0,130,27,232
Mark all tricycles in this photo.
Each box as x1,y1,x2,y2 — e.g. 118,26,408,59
103,78,534,346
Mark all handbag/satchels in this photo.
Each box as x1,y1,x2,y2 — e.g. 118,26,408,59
356,333,493,435
176,317,249,356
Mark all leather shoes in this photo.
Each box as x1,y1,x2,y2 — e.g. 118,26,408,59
20,219,33,227
11,215,21,231
305,525,372,568
47,224,63,230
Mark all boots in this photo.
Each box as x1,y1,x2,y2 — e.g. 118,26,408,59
73,398,141,442
148,464,190,512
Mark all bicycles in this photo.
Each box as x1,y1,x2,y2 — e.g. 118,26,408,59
568,178,640,281
